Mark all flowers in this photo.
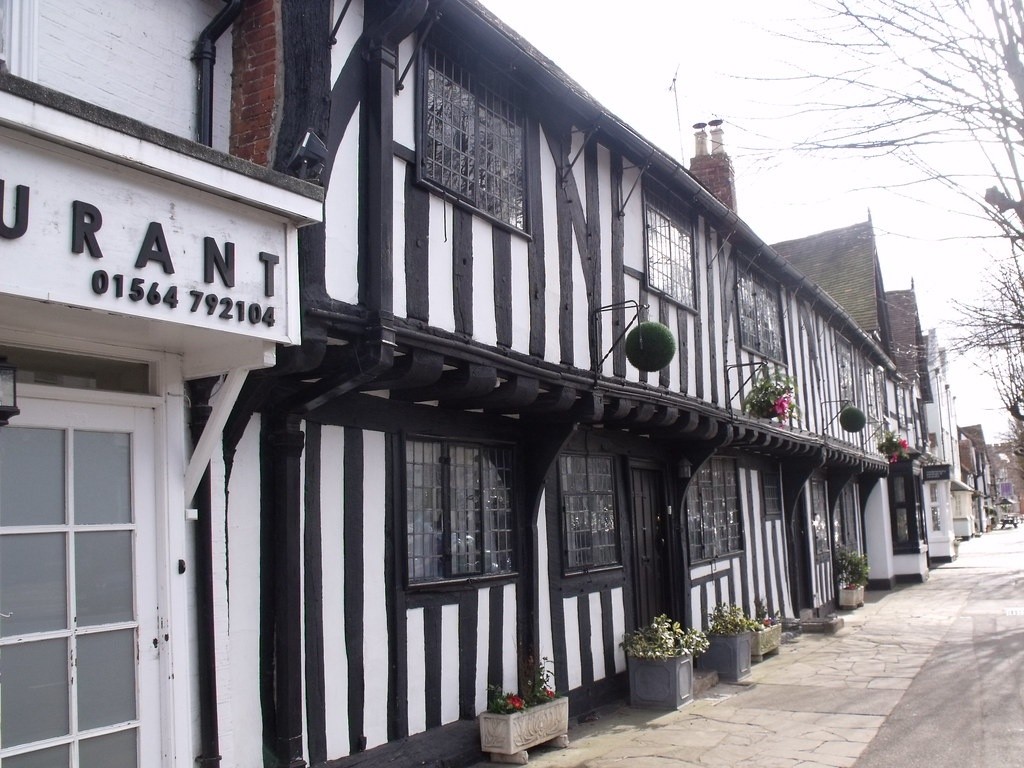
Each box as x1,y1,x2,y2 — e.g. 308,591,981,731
742,365,802,428
485,655,562,714
876,426,909,463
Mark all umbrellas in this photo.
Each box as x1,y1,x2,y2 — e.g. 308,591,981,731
995,498,1013,514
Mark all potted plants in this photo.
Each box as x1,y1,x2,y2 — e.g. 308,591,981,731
697,601,752,682
744,599,782,655
834,548,871,605
619,613,710,711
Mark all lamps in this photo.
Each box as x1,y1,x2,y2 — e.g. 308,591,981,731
0,356,20,425
678,457,692,478
288,128,328,182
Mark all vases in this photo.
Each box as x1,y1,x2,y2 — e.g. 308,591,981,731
480,696,568,755
879,446,897,454
750,400,779,419
